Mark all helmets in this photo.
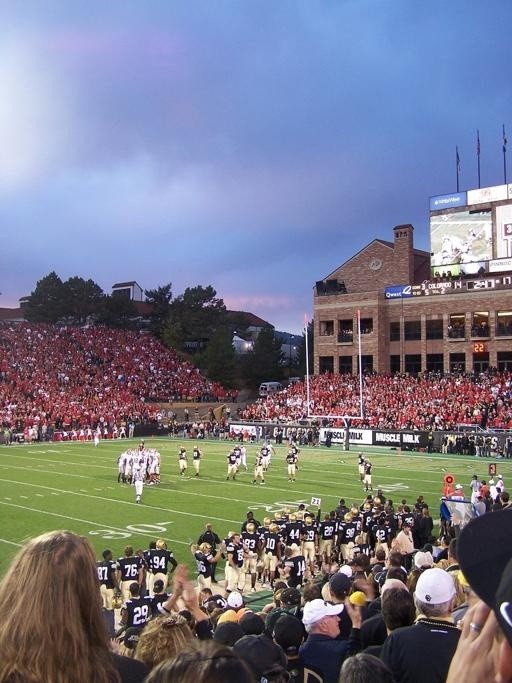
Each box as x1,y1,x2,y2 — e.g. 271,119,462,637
155,540,167,550
344,497,382,520
200,543,212,554
246,507,313,532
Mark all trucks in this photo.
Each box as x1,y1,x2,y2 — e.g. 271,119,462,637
288,375,302,383
258,381,286,398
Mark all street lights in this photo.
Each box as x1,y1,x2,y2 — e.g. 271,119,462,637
289,334,294,377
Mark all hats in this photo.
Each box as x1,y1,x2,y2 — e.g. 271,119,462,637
457,507,512,648
214,587,304,669
302,552,457,632
153,579,164,593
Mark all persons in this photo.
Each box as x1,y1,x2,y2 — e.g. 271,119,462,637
192,444,206,477
114,437,161,503
0,528,151,682
362,457,373,492
226,440,301,485
177,445,189,477
357,451,368,482
441,224,487,264
2,320,240,447
228,321,512,458
434,267,465,276
96,475,512,678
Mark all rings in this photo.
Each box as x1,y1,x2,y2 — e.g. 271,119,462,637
470,623,483,631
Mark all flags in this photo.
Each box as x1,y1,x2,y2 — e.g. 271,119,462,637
453,128,508,176
